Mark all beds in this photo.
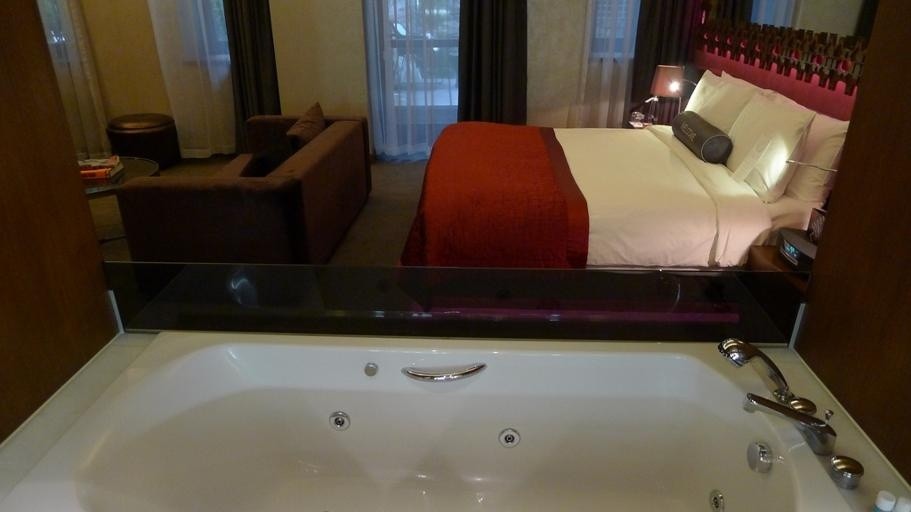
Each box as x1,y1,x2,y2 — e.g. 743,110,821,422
395,25,865,316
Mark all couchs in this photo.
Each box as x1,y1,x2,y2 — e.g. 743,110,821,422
115,110,373,310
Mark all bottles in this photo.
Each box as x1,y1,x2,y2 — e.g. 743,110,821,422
866,489,911,511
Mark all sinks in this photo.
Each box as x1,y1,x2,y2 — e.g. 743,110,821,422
742,394,837,456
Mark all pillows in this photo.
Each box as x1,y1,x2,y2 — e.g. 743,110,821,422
675,66,851,210
286,101,327,151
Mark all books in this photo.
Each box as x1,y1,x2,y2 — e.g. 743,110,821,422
77,155,124,178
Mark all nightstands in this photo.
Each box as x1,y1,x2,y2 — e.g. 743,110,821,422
742,245,808,341
624,118,672,129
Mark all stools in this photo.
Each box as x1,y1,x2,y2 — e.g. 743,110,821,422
106,113,177,170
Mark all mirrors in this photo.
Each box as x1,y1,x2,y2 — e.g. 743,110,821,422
34,1,885,341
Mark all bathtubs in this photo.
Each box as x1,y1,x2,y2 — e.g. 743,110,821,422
0,330,910,512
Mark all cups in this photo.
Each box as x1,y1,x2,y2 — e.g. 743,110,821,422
630,110,643,128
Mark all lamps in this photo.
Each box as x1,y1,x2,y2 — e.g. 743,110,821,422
649,64,697,115
